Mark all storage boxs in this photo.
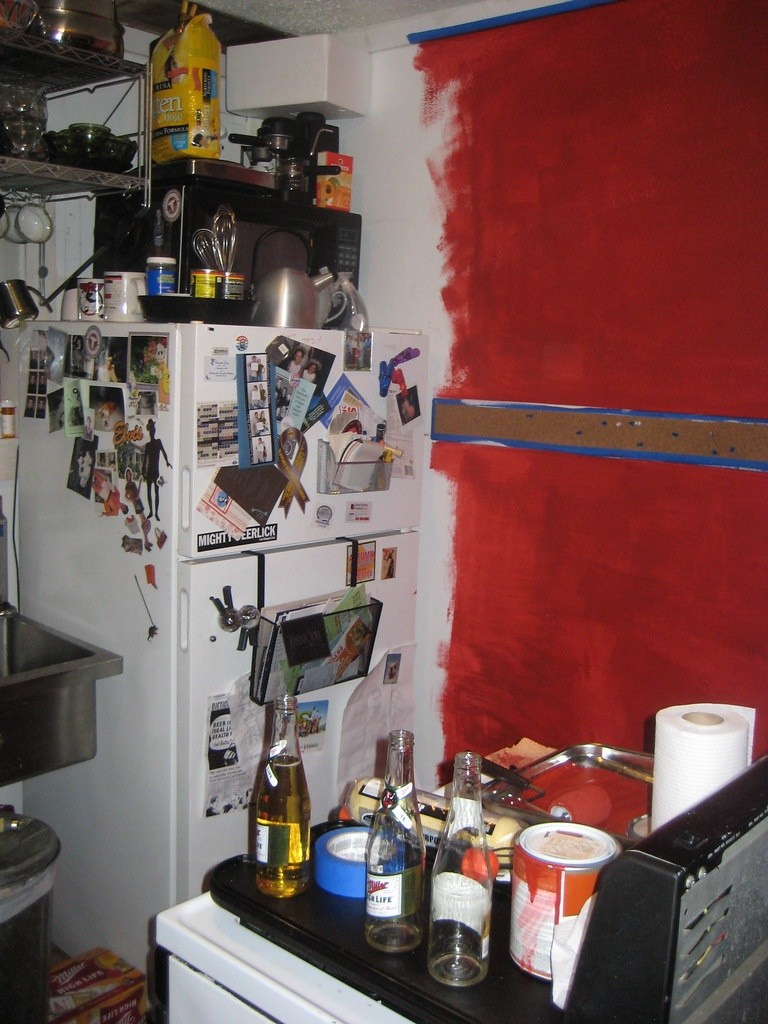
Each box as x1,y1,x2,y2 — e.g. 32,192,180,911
316,150,353,211
48,947,146,1024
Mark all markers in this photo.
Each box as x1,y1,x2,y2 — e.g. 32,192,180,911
374,424,392,488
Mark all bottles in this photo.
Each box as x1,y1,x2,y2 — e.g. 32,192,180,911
254,696,311,898
364,730,427,954
427,747,491,987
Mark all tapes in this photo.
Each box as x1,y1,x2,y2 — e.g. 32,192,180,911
312,826,409,900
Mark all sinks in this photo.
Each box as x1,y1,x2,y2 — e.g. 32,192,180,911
0,606,126,787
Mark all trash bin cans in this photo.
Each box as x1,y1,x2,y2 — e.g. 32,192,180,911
0,813,62,1024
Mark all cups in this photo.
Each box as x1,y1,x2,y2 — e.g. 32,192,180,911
508,823,622,981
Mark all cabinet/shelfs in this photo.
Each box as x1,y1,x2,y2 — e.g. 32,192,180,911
1,25,154,210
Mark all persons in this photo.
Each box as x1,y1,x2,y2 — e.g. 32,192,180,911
84,416,93,440
24,351,48,418
288,347,304,376
275,378,289,421
253,411,269,436
248,356,265,382
256,438,267,463
346,334,371,370
302,358,320,383
250,384,266,409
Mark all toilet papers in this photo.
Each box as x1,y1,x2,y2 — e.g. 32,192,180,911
649,702,756,834
